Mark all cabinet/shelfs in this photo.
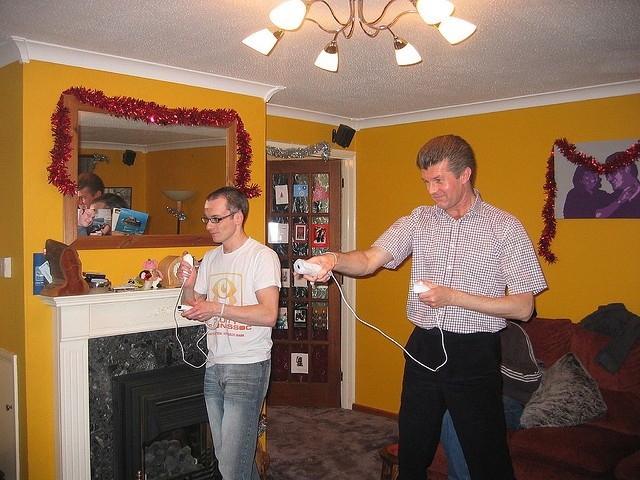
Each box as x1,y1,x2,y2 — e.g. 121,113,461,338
0,348,20,479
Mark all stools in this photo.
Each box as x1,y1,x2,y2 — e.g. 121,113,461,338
380,442,399,480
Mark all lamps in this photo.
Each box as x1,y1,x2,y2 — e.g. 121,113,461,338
242,0,477,72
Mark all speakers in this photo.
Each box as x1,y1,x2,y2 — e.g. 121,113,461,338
333,123,357,149
121,148,137,166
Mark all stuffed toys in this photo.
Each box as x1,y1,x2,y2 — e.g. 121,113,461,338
141,258,165,291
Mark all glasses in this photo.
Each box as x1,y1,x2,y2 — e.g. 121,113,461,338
200,214,232,224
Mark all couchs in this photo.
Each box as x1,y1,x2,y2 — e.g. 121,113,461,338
427,316,640,480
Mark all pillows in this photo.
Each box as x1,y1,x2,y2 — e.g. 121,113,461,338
519,352,608,427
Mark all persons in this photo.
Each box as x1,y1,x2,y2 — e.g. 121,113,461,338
440,320,543,480
78,171,105,210
605,151,640,218
177,187,282,480
90,193,127,216
563,165,612,218
293,135,549,479
78,209,97,236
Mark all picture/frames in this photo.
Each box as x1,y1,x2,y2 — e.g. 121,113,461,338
294,223,308,243
311,224,329,248
62,93,238,250
